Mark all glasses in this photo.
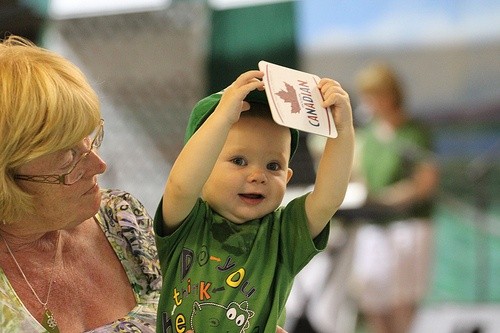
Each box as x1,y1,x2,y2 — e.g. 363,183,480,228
12,119,104,186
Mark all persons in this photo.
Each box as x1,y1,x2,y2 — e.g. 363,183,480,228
0,40,290,333
344,60,446,333
155,70,355,333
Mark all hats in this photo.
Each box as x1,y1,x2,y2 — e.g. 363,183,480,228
184,84,299,164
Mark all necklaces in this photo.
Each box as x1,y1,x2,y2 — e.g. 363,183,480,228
1,231,61,333
